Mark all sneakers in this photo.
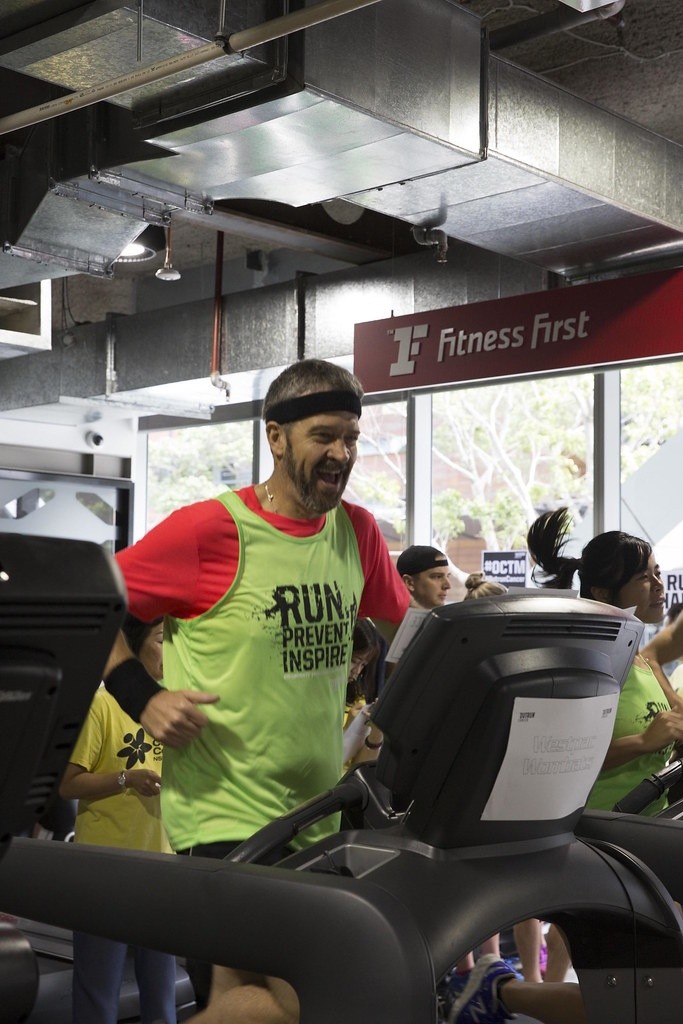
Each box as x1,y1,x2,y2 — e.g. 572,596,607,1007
447,954,522,1024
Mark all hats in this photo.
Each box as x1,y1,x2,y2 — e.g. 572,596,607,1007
397,545,449,576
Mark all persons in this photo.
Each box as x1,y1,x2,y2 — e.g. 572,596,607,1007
99,360,422,1021
449,573,512,998
339,616,383,768
521,505,683,980
57,601,177,1023
443,951,587,1023
371,541,448,702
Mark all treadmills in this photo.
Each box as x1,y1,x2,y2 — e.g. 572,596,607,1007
226,588,683,1024
1,524,428,1022
